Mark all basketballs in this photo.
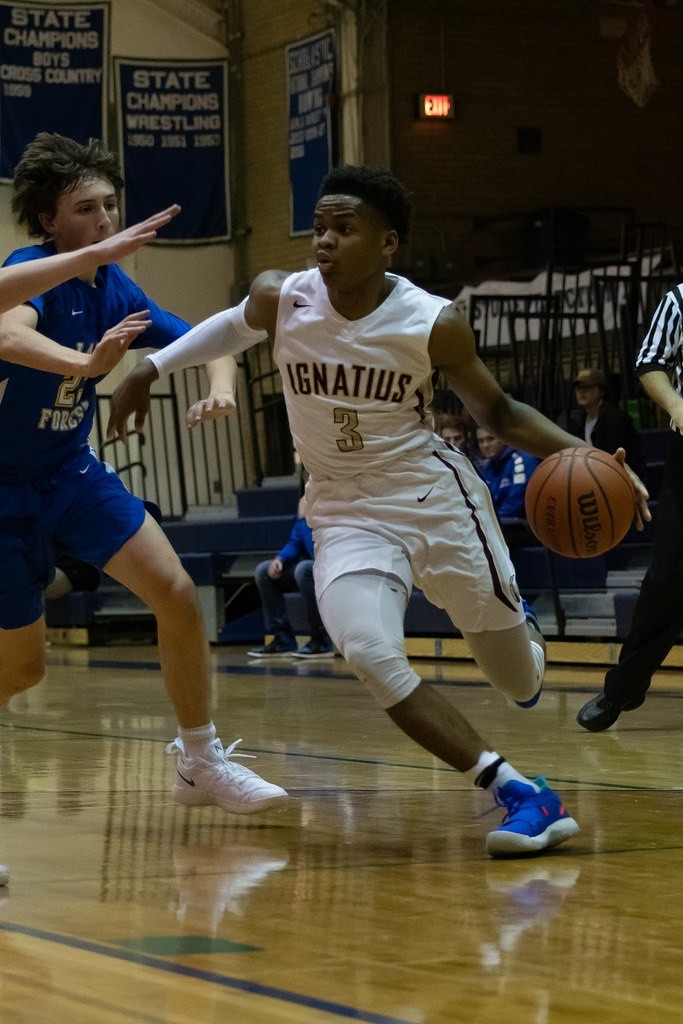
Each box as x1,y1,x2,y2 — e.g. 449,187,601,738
520,447,633,564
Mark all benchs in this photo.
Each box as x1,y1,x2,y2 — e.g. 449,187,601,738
94,427,683,644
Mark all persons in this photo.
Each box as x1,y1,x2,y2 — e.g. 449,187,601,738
0,204,181,888
0,132,292,814
246,497,335,658
571,369,645,478
440,406,482,472
106,166,652,857
475,427,537,547
577,280,683,733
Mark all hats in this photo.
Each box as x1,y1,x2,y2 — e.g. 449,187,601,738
572,368,606,386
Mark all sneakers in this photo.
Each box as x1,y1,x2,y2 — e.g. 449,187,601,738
246,633,298,657
576,690,646,732
515,599,547,708
484,775,580,857
165,735,290,815
291,633,335,658
